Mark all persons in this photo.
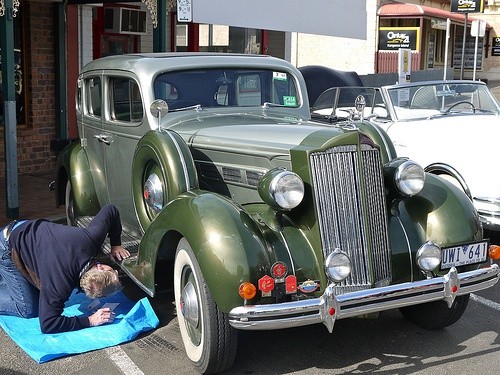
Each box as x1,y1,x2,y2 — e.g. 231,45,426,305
0,205,131,334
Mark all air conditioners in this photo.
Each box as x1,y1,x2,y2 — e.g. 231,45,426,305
105,7,148,36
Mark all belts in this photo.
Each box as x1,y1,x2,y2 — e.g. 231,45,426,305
3,220,18,239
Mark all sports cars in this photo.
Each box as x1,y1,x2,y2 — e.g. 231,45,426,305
298,80,500,233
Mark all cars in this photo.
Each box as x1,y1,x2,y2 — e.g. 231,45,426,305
48,52,500,375
